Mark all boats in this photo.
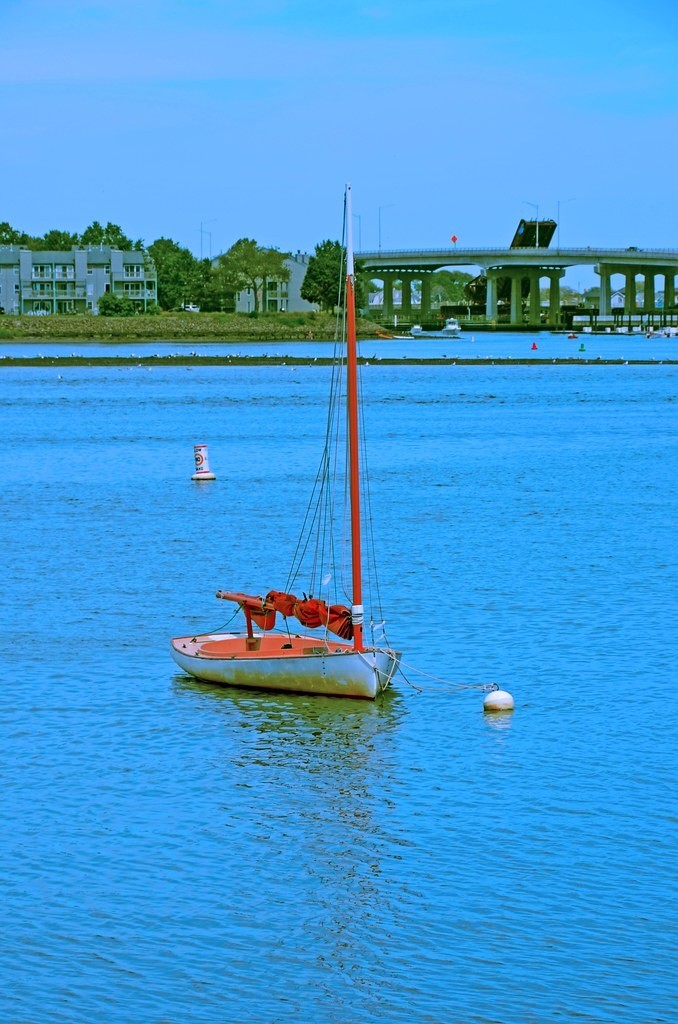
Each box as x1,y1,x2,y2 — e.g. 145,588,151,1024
443,317,462,336
410,324,423,334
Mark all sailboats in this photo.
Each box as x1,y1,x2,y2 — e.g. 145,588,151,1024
167,179,406,700
